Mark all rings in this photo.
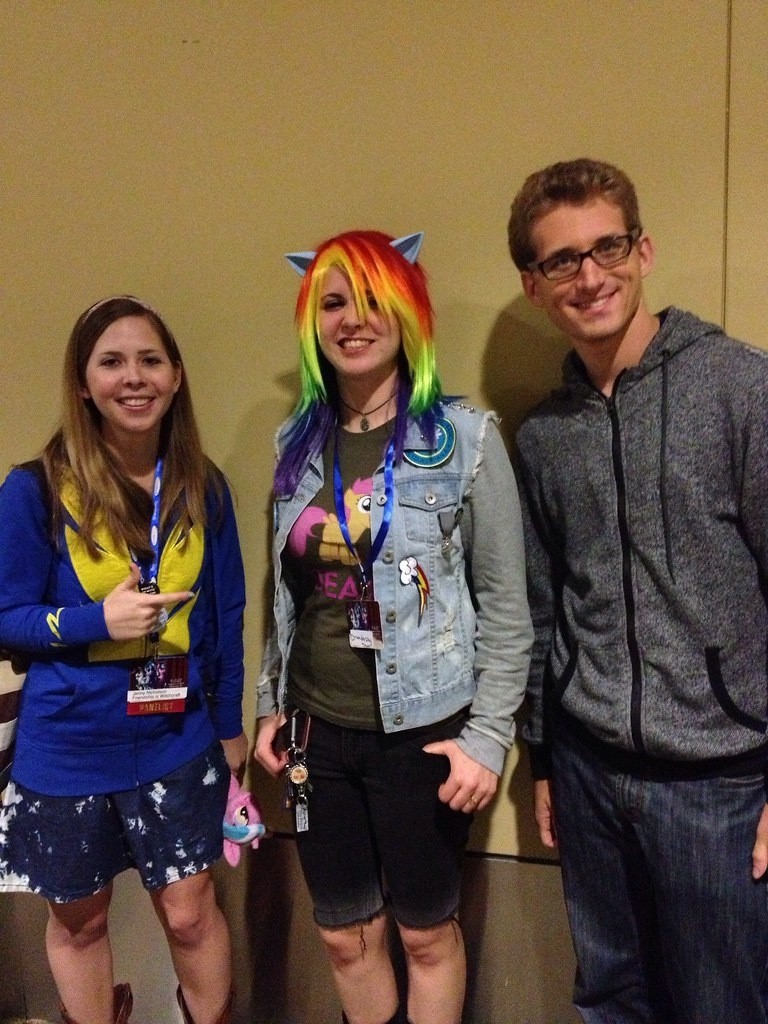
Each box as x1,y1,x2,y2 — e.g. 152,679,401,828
468,797,479,807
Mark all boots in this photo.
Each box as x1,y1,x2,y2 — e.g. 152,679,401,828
59,983,133,1024
175,983,232,1024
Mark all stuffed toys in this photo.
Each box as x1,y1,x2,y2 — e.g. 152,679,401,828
221,775,264,867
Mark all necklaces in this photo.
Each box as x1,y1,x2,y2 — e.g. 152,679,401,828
337,391,398,430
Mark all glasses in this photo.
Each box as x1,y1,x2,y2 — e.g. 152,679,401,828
527,226,641,281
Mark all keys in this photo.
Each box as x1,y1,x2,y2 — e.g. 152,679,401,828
283,705,312,834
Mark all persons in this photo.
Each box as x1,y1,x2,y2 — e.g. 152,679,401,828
0,296,247,1024
506,159,768,1024
253,231,533,1024
